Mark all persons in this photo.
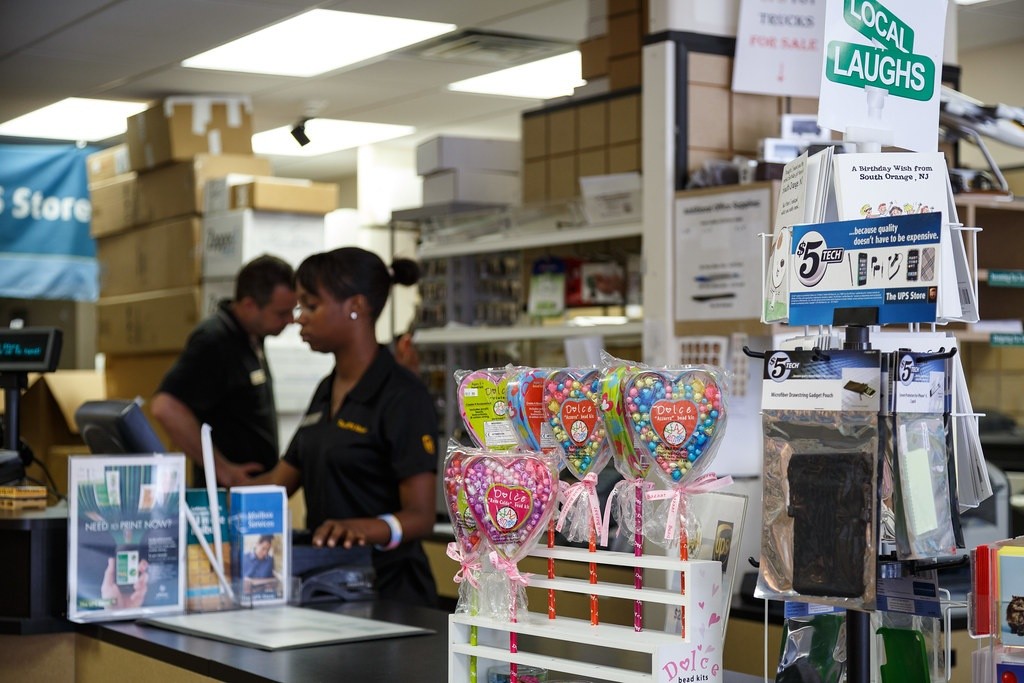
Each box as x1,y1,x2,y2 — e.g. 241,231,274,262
252,247,441,612
150,256,298,488
244,535,274,578
100,558,149,610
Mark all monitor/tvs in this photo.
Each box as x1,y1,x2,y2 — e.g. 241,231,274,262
74,399,163,454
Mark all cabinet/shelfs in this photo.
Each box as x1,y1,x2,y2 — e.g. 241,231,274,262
900,191,1023,344
386,189,645,567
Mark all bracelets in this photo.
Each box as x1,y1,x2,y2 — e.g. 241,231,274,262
375,514,402,551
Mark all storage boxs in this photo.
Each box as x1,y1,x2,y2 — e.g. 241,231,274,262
86,96,341,495
414,0,814,204
